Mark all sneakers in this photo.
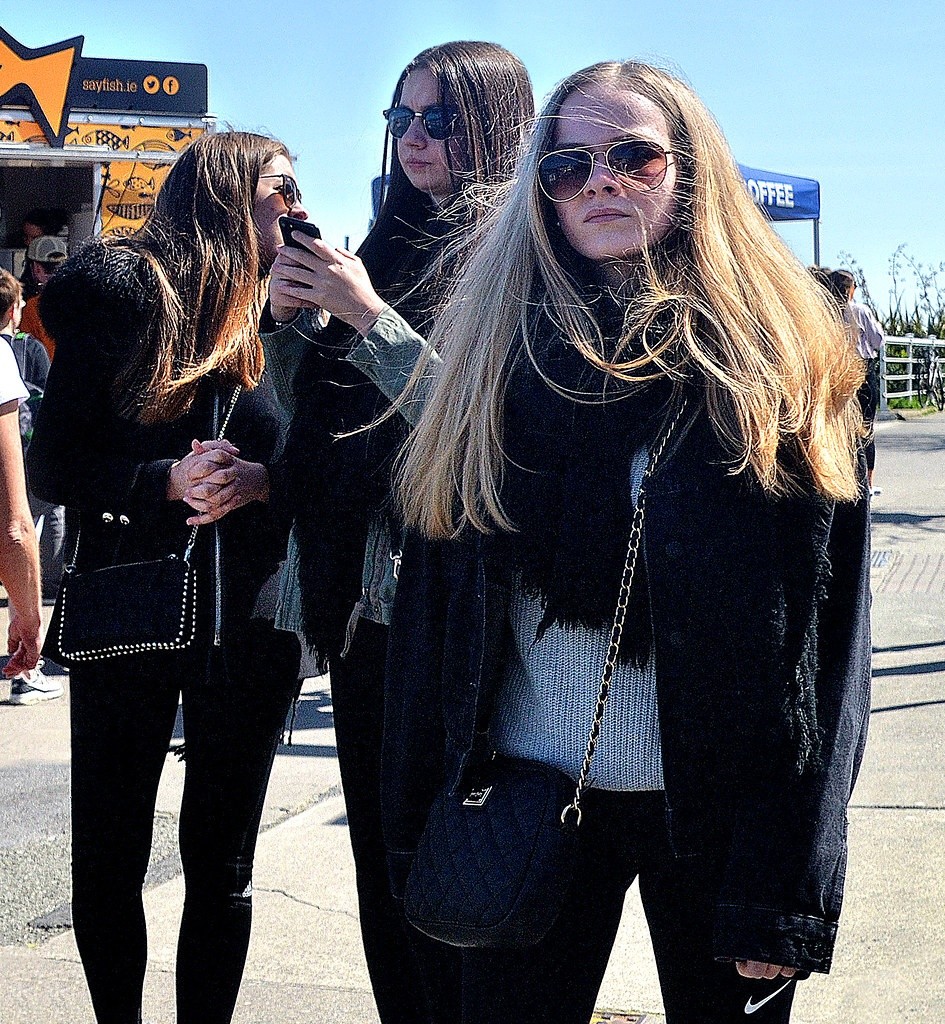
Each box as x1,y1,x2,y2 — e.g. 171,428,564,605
9,659,64,705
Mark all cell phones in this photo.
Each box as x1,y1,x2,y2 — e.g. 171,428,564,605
277,217,324,288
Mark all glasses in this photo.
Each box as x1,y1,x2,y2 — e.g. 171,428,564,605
19,299,26,307
258,173,302,209
536,140,688,203
383,101,463,140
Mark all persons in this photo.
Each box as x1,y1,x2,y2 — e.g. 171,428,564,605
0,198,94,710
262,32,542,1024
30,127,330,1024
421,59,876,1024
807,268,881,480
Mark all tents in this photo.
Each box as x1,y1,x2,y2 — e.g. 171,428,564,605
724,156,824,275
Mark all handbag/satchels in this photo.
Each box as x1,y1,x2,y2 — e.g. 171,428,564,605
401,760,590,953
57,558,207,661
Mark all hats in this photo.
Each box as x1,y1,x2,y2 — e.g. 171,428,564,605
27,236,68,262
